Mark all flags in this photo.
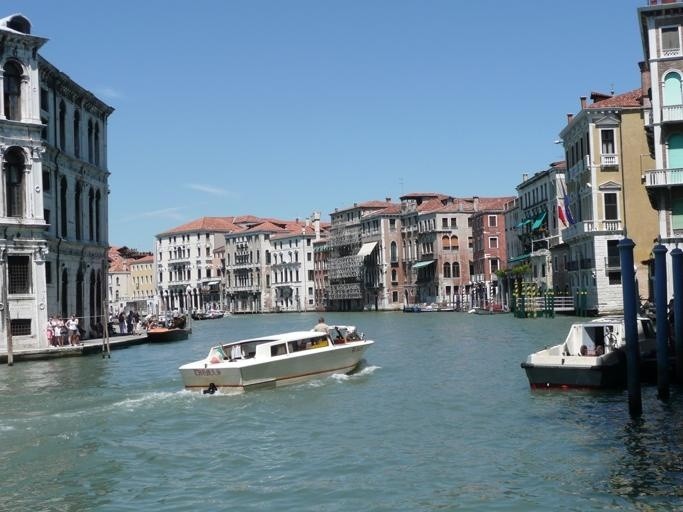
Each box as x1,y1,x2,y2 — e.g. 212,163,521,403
557,206,567,227
564,195,575,224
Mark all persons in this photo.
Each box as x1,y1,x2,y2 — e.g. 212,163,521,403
578,345,588,355
313,318,331,343
595,344,604,356
47,302,225,347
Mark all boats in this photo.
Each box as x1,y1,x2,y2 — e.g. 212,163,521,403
521,314,657,393
179,325,374,394
403,305,454,312
147,308,223,341
467,307,509,314
